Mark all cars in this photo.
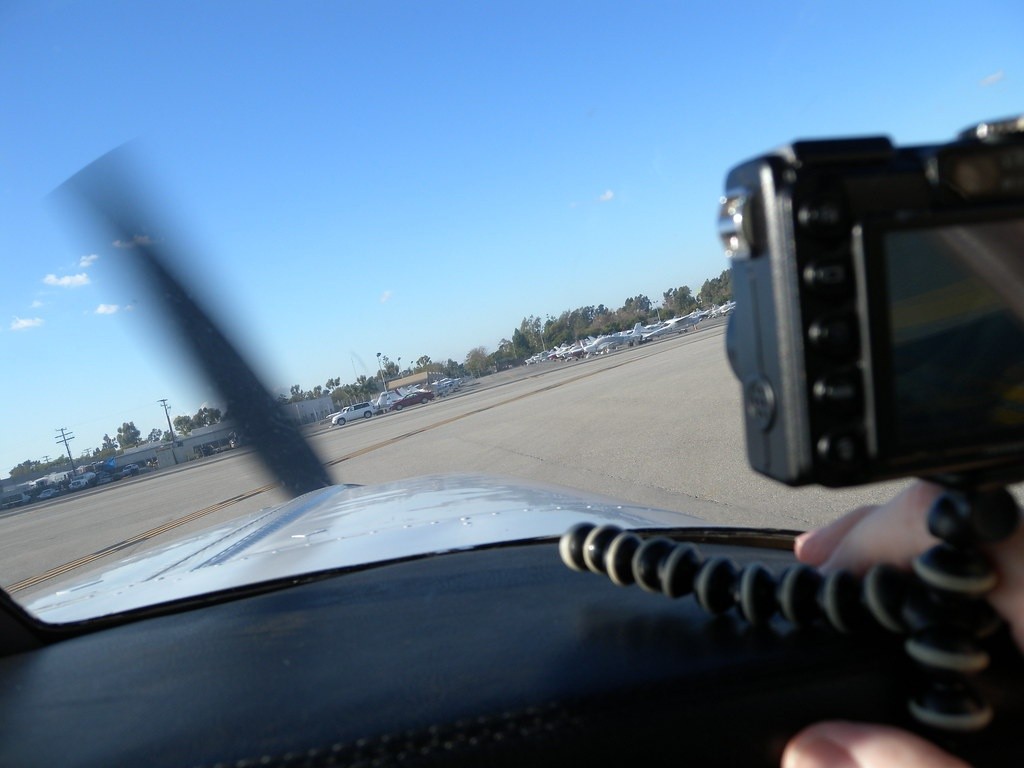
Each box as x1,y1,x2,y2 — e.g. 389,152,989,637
389,391,434,411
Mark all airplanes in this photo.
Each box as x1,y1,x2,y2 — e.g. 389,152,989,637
325,378,461,418
525,301,736,364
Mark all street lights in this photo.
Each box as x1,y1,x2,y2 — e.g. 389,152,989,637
653,300,661,321
398,357,403,379
377,353,388,393
411,361,415,375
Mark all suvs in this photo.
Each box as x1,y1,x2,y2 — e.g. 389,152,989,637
122,464,139,477
332,401,376,426
198,446,220,457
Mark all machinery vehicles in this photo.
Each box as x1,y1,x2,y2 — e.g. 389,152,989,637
68,472,97,490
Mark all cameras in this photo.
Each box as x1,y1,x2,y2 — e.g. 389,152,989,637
714,113,1023,488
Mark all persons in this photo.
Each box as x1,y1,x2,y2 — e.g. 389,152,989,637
229,430,238,449
492,360,513,373
781,478,1024,768
63,475,73,494
147,459,160,471
